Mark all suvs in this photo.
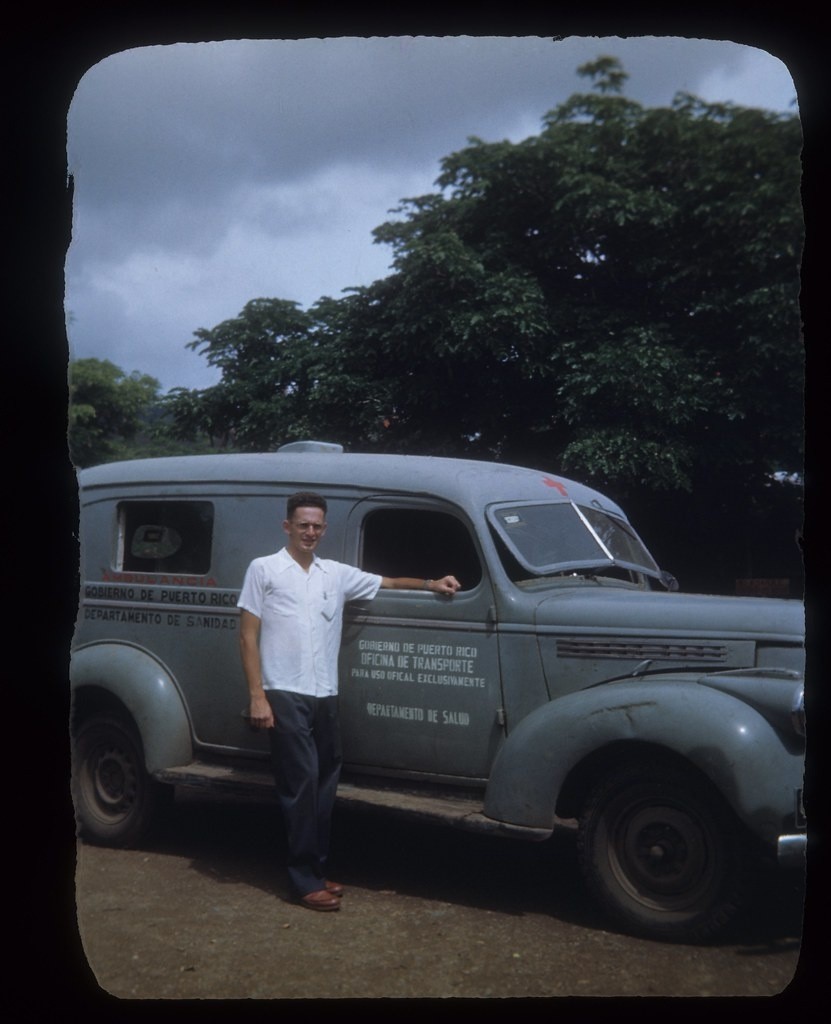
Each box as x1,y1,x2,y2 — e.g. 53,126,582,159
73,441,810,933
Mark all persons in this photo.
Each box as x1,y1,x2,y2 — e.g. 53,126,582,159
236,492,462,911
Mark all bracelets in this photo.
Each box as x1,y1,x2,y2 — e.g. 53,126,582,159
424,579,434,591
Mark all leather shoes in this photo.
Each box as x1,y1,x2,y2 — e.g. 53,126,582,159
324,880,344,896
288,889,341,911
